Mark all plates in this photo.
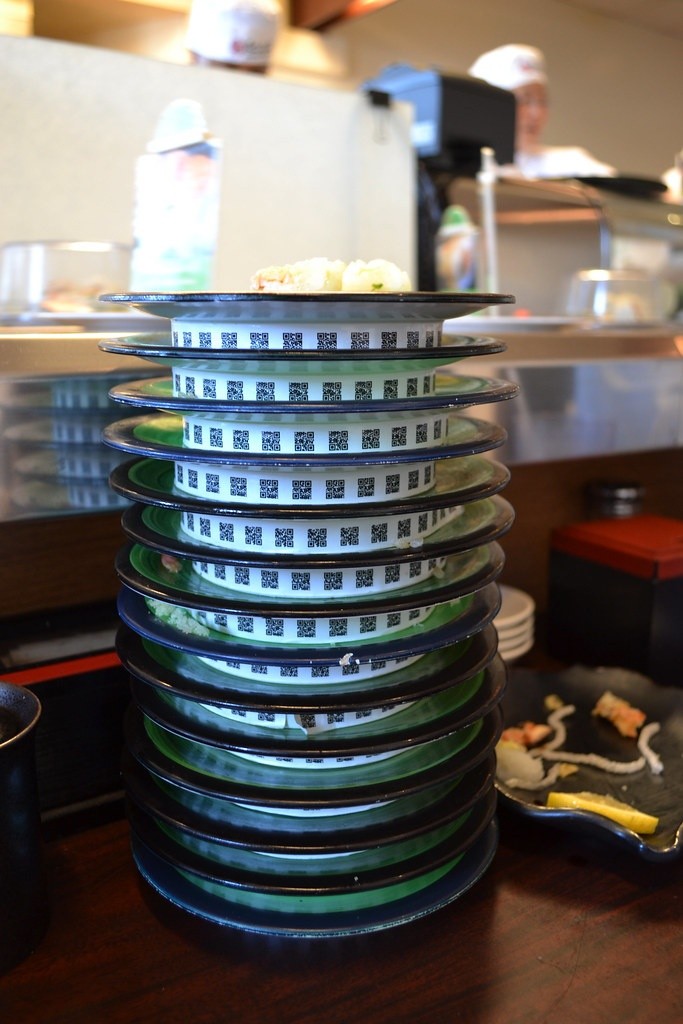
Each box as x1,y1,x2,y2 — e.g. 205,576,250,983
443,315,585,336
493,643,683,865
99,289,516,941
0,307,172,332
493,582,534,665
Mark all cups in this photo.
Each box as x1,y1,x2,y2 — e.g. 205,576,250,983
0,679,48,977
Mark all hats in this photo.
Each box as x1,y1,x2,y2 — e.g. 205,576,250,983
188,0,279,65
467,44,547,91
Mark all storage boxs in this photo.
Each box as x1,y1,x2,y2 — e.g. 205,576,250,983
547,514,683,684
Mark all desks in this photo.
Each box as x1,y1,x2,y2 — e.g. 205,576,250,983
0,648,683,1024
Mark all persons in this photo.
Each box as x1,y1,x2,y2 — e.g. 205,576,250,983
469,42,617,176
187,0,279,71
